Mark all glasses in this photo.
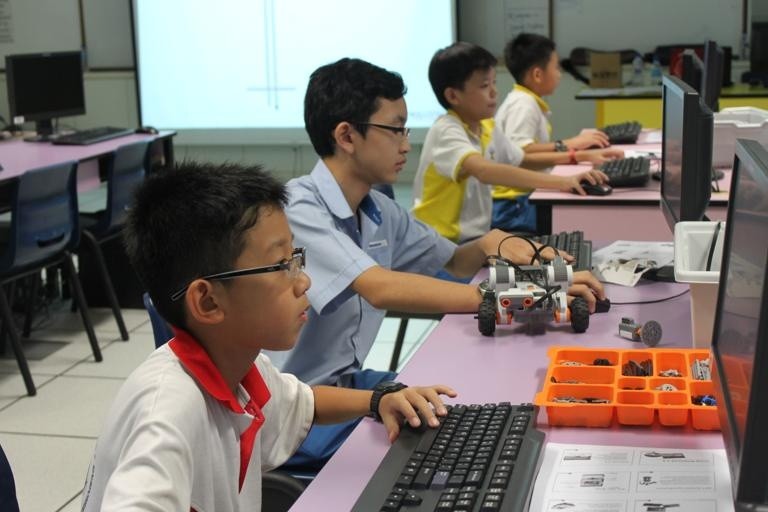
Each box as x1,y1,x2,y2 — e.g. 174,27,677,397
171,247,307,301
350,122,411,139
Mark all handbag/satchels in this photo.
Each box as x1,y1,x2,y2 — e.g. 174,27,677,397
560,48,643,88
644,43,705,65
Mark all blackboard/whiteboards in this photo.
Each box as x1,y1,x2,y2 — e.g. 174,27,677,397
77,0,555,77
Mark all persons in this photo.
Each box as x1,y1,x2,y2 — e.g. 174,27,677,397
488,29,611,230
406,39,625,283
242,57,607,469
78,158,458,512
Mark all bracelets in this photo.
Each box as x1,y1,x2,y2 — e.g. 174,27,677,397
555,140,568,152
569,145,579,165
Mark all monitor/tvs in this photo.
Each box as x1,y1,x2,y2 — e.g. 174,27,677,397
708,138,768,504
641,75,714,282
705,39,724,110
681,50,706,100
5,50,86,141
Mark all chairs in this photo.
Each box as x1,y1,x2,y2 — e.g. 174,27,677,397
378,185,445,372
142,292,305,511
0,445,20,512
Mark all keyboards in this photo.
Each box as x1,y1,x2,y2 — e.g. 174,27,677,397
350,403,544,512
593,156,650,188
52,127,134,145
526,228,593,272
598,121,641,144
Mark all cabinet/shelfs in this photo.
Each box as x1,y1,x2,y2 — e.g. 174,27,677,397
0,0,139,126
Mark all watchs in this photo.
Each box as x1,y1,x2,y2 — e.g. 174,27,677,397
368,379,409,424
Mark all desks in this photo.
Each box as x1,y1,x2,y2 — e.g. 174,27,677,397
290,266,734,512
528,128,768,252
575,83,768,128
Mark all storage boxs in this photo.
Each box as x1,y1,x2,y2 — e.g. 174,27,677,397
589,53,622,88
674,221,727,349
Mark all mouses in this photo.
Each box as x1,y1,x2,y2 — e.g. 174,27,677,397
589,142,607,150
571,290,611,314
575,183,612,196
136,126,158,135
